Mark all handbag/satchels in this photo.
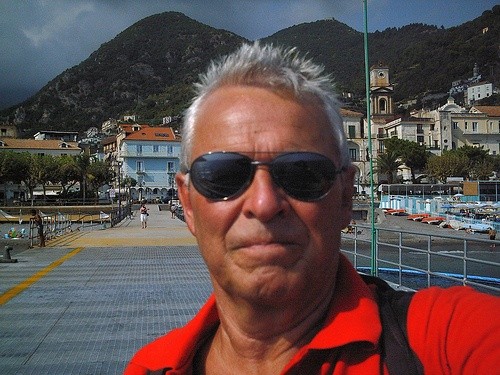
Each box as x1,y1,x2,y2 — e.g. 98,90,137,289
147,213,149,216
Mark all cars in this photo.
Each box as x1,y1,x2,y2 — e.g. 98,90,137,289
168,199,182,209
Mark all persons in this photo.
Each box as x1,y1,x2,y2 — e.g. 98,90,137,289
34,215,45,247
124,42,500,375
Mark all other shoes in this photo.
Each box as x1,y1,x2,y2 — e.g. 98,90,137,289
142,226,147,229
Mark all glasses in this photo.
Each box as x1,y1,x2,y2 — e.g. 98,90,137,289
183,150,343,203
